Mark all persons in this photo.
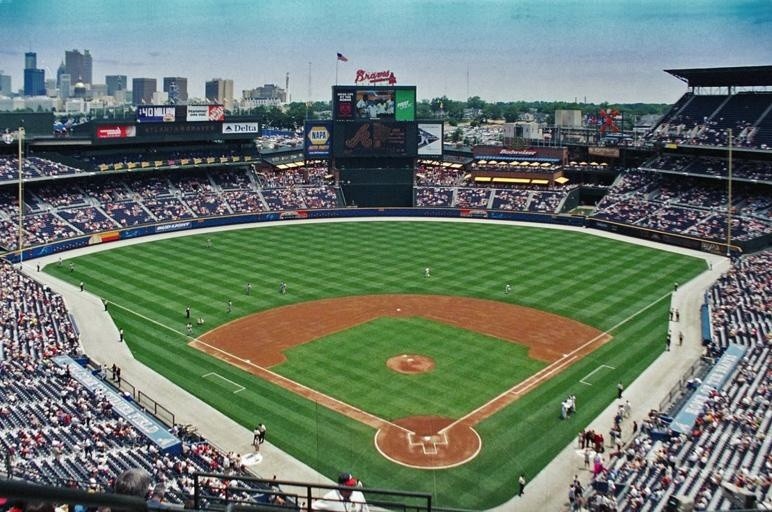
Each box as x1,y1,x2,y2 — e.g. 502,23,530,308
567,395,573,415
357,93,369,117
366,100,379,117
376,98,388,118
308,472,370,512
423,266,431,279
504,280,511,295
386,96,394,117
561,398,570,419
517,472,527,498
356,479,363,487
299,501,305,512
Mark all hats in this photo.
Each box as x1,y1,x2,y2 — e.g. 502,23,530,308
338,472,356,487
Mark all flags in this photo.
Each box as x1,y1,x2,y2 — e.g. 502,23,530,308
336,53,348,62
